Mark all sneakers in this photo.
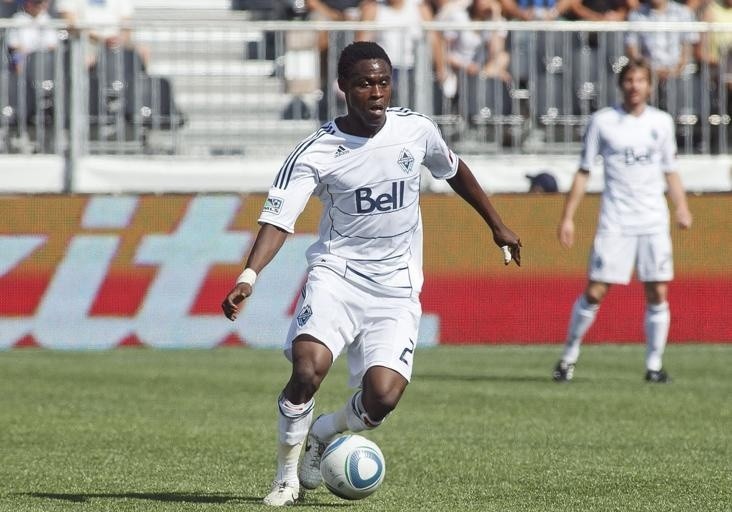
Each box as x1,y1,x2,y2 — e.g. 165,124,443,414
297,414,335,488
553,359,576,382
263,478,303,506
645,369,670,384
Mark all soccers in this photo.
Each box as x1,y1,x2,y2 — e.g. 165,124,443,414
320,436,384,501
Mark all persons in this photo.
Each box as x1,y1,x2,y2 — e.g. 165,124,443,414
217,39,525,508
524,172,560,192
0,0,187,156
278,0,732,158
550,55,694,381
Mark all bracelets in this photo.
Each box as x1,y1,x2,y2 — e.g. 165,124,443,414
235,268,258,288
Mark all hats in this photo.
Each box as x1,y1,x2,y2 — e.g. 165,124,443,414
525,172,555,193
